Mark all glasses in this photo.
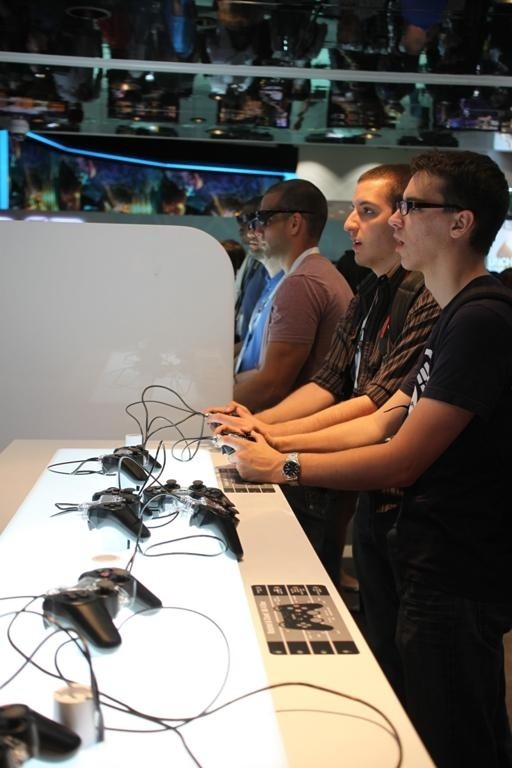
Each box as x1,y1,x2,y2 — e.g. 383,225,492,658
393,196,465,217
255,209,314,224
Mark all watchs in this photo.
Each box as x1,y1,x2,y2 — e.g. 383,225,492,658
281,451,301,489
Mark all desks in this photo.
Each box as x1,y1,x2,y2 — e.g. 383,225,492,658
1,437,438,768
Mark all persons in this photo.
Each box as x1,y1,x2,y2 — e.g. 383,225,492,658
205,159,439,666
230,178,353,413
0,1,511,376
210,146,512,768
